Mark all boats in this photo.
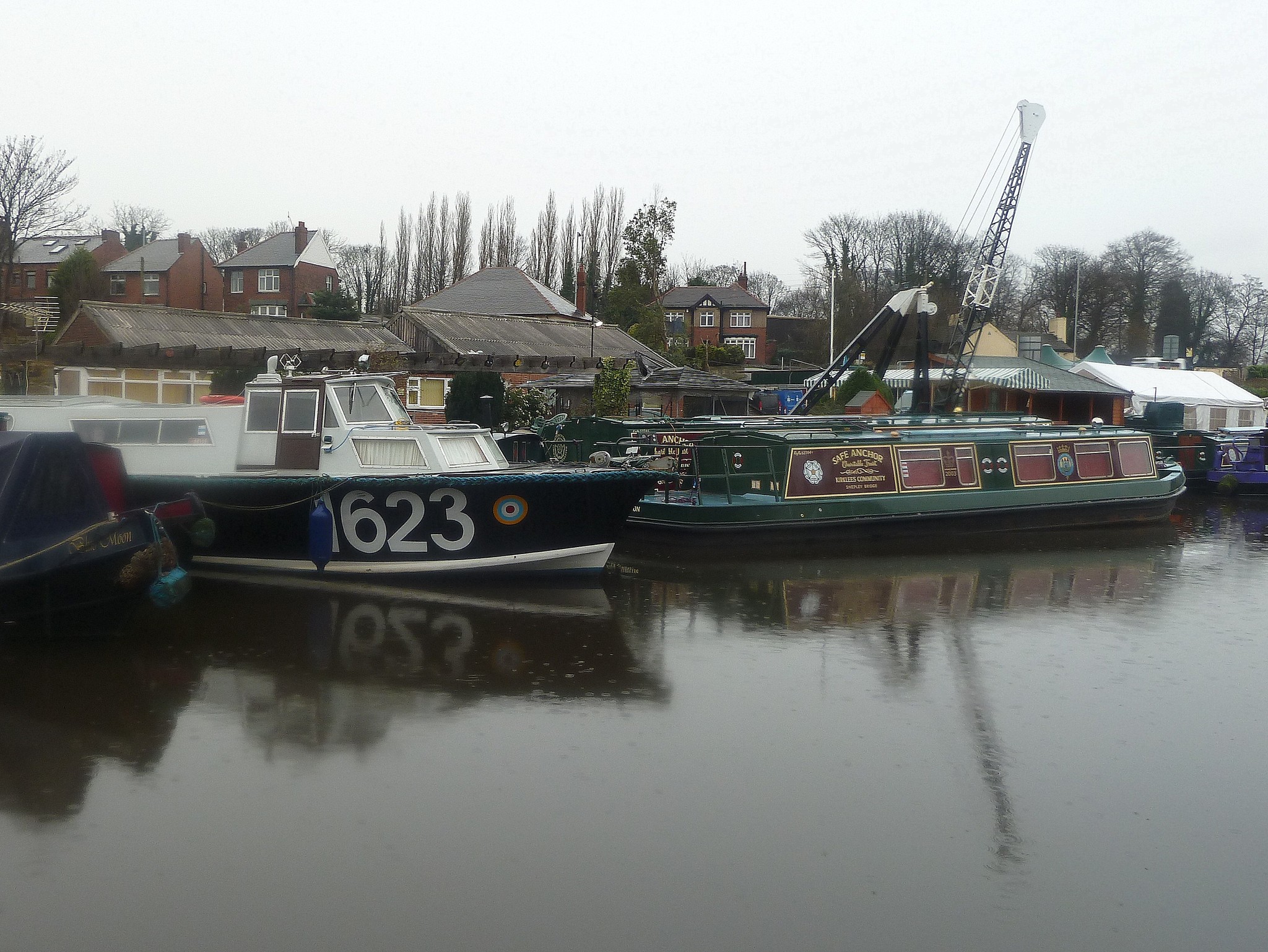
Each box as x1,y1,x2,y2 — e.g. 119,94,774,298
1,429,205,640
623,415,1268,550
18,345,663,592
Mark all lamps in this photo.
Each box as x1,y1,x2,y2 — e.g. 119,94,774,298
500,422,509,438
358,354,370,373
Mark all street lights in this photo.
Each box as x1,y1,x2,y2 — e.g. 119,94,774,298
1073,252,1080,368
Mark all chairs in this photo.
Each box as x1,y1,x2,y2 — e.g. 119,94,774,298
530,413,567,432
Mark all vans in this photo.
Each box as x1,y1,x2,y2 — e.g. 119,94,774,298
749,390,804,415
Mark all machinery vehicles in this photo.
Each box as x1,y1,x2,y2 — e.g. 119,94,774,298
788,99,1046,411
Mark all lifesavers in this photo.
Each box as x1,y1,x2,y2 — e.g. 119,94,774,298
199,395,245,404
